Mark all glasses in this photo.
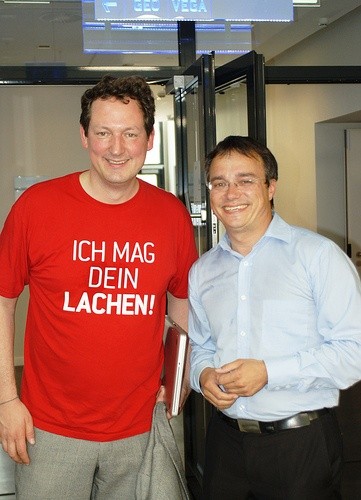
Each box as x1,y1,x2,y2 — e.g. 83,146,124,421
206,176,266,190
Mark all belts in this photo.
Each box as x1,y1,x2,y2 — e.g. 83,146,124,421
217,408,325,435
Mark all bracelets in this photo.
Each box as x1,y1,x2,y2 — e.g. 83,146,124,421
0,395,19,405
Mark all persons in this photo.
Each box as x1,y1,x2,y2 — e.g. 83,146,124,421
187,134,361,500
0,74,200,500
